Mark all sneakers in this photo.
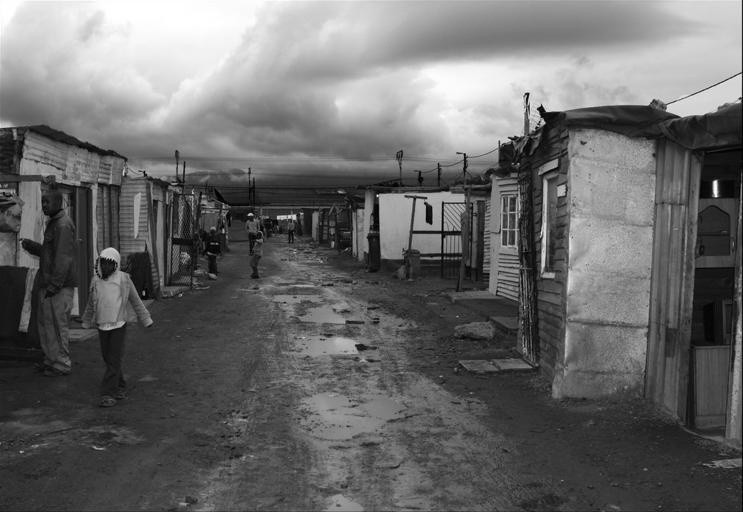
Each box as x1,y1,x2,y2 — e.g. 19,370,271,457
32,361,62,376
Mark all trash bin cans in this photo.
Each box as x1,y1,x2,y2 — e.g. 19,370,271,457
404,249,420,281
367,233,380,271
128,252,153,300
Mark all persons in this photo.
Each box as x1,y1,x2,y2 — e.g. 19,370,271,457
202,226,220,276
19,189,81,377
244,213,295,279
81,247,154,408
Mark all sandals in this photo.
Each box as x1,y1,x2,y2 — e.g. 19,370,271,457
99,392,129,407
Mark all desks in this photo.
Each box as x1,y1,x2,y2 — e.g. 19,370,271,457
691,341,731,430
